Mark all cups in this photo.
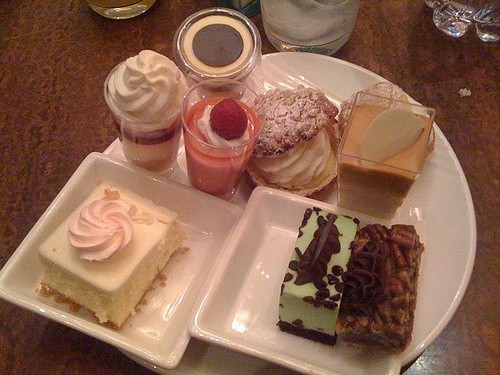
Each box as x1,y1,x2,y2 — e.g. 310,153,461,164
103,61,189,179
216,0,261,18
87,0,155,19
180,78,265,202
424,0,500,42
259,0,359,56
337,91,436,220
173,7,261,101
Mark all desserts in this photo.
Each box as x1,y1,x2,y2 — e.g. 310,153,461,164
337,81,412,137
337,223,425,354
181,14,256,98
38,182,187,329
246,84,343,197
276,206,359,346
181,94,259,196
104,48,189,133
336,102,432,221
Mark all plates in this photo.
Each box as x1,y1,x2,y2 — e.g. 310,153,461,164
102,52,477,375
0,151,244,371
